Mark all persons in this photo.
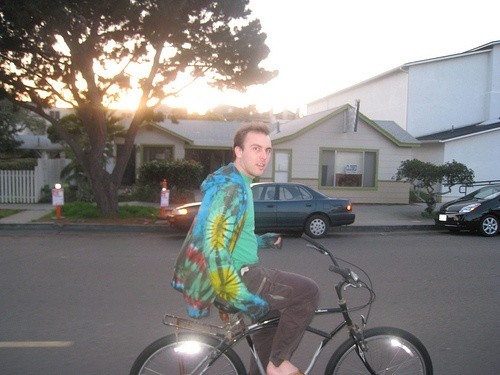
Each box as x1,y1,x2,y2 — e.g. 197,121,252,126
171,121,320,375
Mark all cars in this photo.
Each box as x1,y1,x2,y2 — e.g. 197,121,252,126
168,182,356,239
435,183,500,238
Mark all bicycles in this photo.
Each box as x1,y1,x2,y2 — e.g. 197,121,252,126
129,234,433,375
